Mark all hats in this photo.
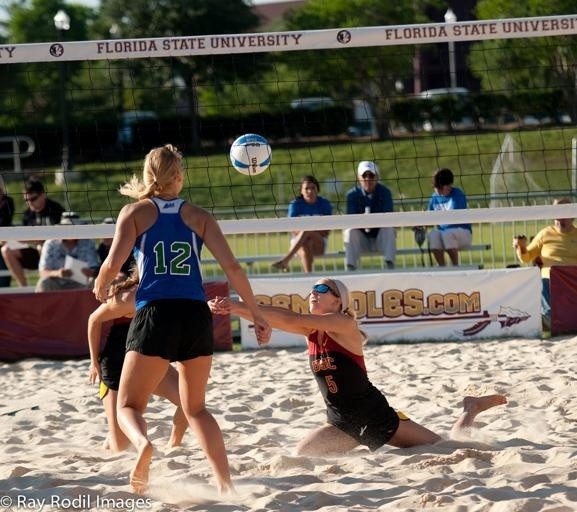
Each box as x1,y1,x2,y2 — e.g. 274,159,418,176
59,212,80,225
431,168,453,187
357,161,376,175
331,278,349,313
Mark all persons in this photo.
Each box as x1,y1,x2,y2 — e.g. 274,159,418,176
511,196,576,330
341,160,397,273
270,174,332,274
83,259,189,455
30,211,100,293
0,179,67,289
203,275,508,461
0,174,14,288
89,144,274,504
414,167,473,266
87,215,134,280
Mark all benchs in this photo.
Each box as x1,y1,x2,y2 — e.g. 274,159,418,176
0,244,492,296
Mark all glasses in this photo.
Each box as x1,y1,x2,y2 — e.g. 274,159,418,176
313,284,339,298
25,195,38,202
362,173,373,178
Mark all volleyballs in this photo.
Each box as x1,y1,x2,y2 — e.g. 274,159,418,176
228,133,273,177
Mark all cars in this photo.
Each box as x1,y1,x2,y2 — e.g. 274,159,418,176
292,84,473,140
115,109,156,160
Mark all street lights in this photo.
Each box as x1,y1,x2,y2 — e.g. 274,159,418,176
444,8,458,88
53,8,74,171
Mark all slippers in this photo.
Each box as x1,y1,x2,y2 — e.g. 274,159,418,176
271,261,285,269
